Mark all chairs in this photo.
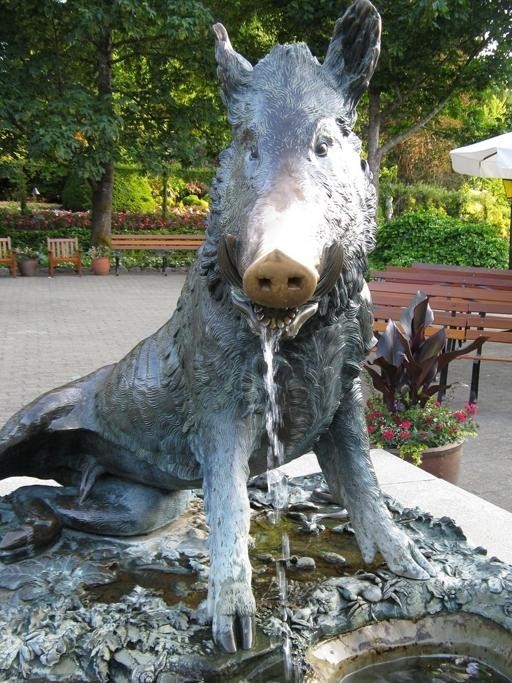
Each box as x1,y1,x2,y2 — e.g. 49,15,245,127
0,236,17,278
47,236,84,278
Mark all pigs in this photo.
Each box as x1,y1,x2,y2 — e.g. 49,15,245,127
0,0,437,659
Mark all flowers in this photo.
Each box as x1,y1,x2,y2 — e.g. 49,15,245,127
363,289,489,466
87,245,112,261
15,245,39,264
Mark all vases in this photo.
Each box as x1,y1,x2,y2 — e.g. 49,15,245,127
92,257,111,275
18,257,39,277
370,439,463,489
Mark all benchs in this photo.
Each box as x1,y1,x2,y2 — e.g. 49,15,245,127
365,261,511,409
111,235,206,277
